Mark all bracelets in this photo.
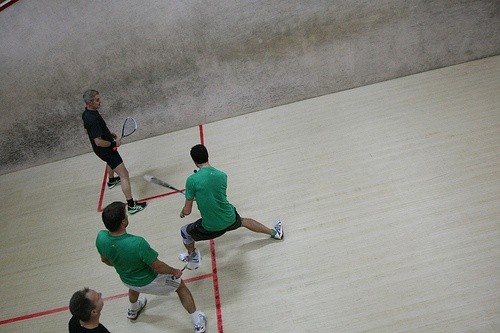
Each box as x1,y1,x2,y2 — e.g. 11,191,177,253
109,141,116,147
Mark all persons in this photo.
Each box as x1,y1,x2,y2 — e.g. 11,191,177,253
82,91,148,215
178,143,284,263
68,287,110,333
96,201,207,333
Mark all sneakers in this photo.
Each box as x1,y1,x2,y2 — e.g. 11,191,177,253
273,220,283,240
193,311,206,333
179,252,203,263
127,200,147,214
127,297,147,321
106,176,120,189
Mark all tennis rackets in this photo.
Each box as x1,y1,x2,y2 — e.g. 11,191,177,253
143,174,186,196
112,116,138,152
